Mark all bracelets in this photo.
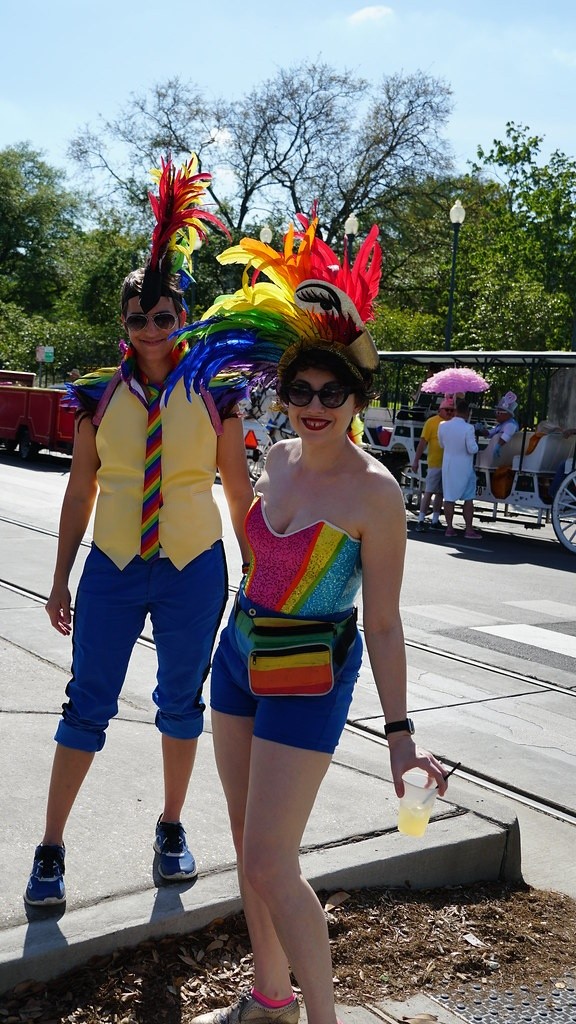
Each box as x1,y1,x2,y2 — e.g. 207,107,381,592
242,563,249,573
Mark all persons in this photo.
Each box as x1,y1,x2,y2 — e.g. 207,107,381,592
475,397,518,467
438,397,482,538
69,369,79,381
412,399,454,532
25,268,253,906
191,351,447,1024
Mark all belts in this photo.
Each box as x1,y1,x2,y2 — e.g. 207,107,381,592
136,547,169,558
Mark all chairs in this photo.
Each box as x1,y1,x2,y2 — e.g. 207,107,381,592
397,393,429,420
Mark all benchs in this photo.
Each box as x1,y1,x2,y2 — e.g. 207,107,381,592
474,432,576,474
366,407,393,428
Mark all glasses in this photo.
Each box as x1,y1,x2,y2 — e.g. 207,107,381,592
441,407,454,412
282,380,355,409
124,312,179,333
494,411,508,416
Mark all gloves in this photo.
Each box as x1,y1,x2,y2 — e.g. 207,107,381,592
492,442,500,460
475,422,484,432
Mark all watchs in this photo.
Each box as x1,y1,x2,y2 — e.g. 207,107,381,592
384,719,415,736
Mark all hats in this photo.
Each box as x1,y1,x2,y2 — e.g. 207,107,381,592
495,391,518,417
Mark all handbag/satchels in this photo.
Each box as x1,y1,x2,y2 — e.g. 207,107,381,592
233,589,358,696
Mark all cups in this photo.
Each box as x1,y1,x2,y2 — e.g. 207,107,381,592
397,772,439,836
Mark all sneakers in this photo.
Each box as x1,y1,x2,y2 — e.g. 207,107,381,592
190,992,300,1024
152,813,197,880
416,521,425,532
465,531,482,539
445,529,457,536
23,841,66,905
429,520,447,531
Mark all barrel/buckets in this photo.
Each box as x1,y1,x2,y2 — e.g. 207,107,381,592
379,430,391,446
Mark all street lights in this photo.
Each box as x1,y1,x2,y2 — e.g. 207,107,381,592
445,199,466,349
345,212,359,265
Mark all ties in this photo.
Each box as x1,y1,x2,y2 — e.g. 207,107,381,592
140,372,169,565
